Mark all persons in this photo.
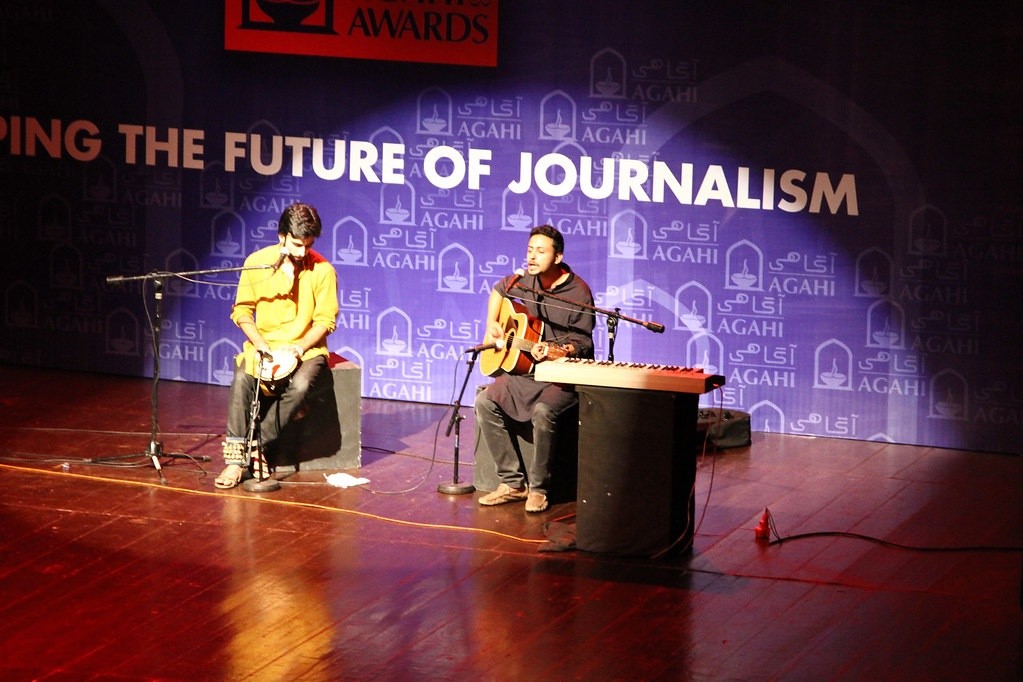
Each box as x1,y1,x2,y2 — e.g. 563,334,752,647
474,225,596,511
214,204,340,489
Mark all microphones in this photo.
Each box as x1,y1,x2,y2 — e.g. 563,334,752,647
256,349,273,362
466,338,504,354
273,246,290,271
505,266,526,295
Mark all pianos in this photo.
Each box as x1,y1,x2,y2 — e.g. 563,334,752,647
533,354,727,394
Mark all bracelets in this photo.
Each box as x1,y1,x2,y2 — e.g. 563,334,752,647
293,346,304,358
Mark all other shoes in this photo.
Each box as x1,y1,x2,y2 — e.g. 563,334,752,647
248,440,270,480
478,483,528,506
525,490,551,512
214,464,243,489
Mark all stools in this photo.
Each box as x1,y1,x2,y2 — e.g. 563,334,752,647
268,352,362,472
473,387,579,492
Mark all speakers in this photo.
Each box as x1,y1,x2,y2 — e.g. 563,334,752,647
573,385,702,561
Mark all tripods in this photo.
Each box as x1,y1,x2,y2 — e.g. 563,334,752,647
83,263,273,488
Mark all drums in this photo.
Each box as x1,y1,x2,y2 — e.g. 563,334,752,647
257,351,312,422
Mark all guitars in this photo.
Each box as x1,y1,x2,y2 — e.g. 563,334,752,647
477,296,570,378
